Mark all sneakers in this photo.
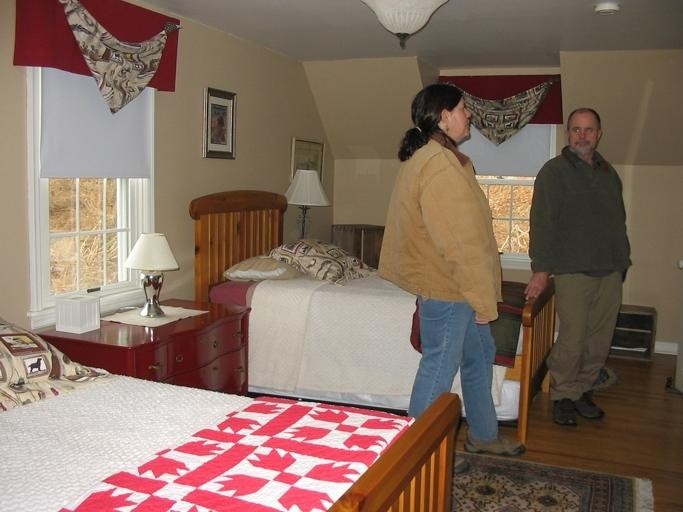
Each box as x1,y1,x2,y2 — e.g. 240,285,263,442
465,436,525,455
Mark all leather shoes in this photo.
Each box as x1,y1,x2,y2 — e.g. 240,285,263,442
554,399,577,427
579,394,604,417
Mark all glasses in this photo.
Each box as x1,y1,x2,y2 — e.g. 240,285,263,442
571,127,598,135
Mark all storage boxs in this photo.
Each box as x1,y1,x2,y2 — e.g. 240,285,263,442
608,305,657,362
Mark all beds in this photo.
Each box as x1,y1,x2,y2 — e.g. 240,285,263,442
0,375,462,512
188,190,557,445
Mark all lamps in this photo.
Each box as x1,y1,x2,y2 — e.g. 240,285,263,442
283,170,333,240
123,233,180,318
361,0,450,47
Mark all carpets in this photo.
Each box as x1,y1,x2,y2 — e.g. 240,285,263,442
451,450,654,512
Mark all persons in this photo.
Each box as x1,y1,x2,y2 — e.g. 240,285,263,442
376,83,528,457
523,108,632,427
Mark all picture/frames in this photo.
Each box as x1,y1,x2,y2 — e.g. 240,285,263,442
203,86,237,160
291,136,326,185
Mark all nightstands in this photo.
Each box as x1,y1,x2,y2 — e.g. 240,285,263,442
37,298,252,396
